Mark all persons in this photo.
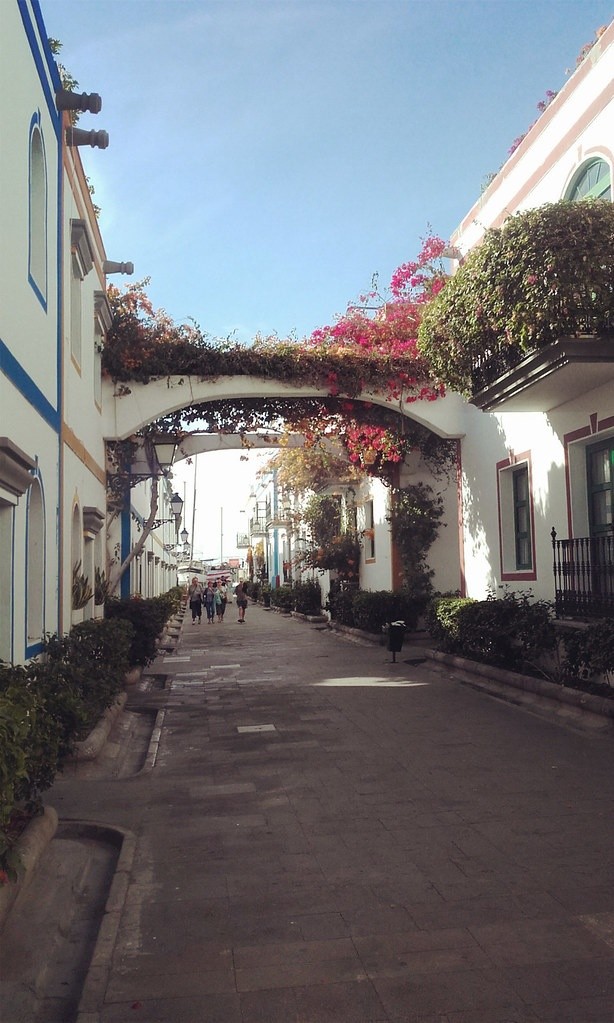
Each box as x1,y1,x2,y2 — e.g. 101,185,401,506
235,580,248,623
188,577,204,625
203,578,228,624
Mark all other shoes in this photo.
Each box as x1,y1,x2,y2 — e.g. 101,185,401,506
192,620,196,625
218,620,221,623
222,619,224,622
238,619,245,623
197,620,201,624
212,622,215,624
208,621,211,624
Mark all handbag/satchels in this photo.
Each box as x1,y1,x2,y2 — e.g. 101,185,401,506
242,582,248,592
190,599,192,609
220,591,228,604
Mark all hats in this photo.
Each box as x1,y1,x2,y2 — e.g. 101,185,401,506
239,577,244,582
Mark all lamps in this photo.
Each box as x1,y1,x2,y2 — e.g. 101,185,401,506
106,426,181,500
171,540,190,561
165,528,189,551
360,446,393,488
143,492,185,532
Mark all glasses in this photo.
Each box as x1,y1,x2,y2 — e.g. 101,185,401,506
217,582,221,583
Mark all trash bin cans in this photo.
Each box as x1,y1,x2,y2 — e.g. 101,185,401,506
384,619,408,653
261,591,271,607
